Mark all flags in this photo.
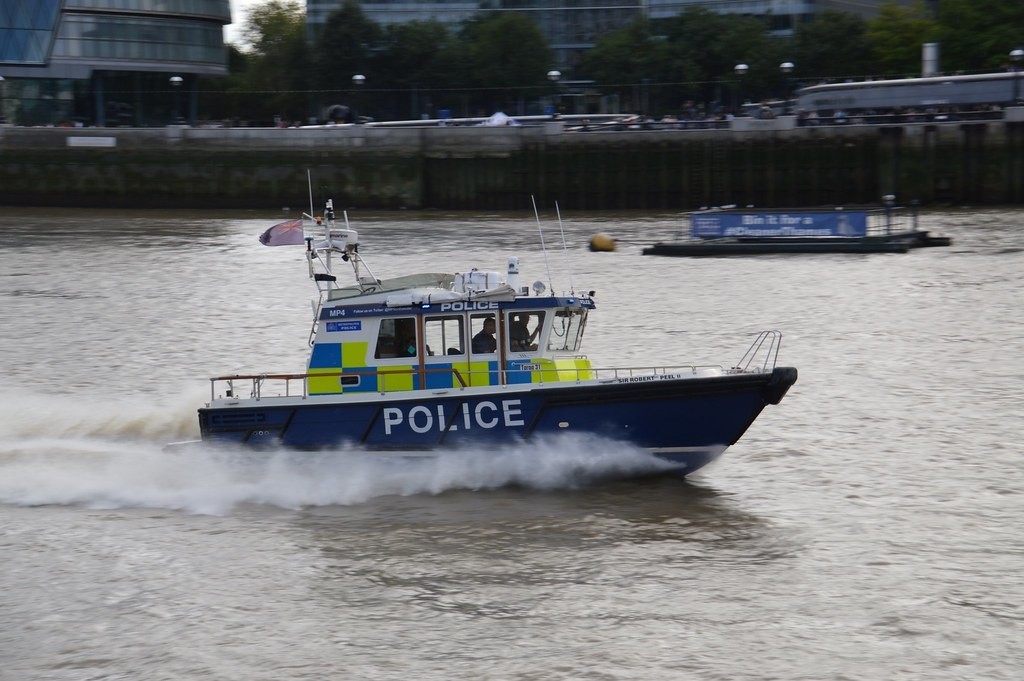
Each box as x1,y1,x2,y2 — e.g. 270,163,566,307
259,219,305,247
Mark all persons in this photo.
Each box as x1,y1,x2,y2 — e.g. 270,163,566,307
47,112,317,129
398,327,431,360
472,318,497,355
578,98,1002,132
509,314,543,351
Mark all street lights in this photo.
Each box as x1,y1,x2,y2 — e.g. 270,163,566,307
734,64,749,119
351,75,365,126
779,63,796,111
169,75,184,127
546,70,564,125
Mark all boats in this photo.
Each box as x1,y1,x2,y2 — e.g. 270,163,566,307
640,191,959,256
159,159,799,476
